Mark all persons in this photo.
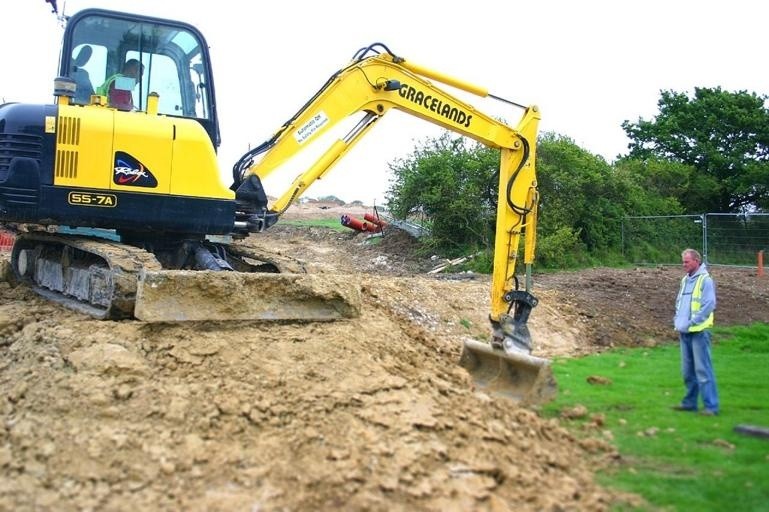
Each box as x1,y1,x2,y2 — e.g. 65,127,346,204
672,248,719,416
96,58,145,111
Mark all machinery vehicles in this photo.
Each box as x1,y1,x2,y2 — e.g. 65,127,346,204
0,1,559,410
